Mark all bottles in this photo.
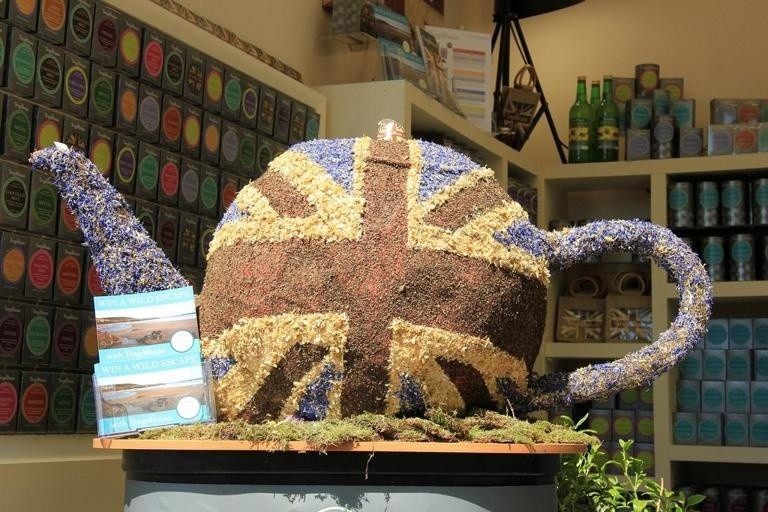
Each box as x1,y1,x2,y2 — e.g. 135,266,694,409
568,72,596,164
589,73,621,162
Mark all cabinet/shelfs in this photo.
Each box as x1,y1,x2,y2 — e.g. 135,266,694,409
322,80,541,226
541,153,768,511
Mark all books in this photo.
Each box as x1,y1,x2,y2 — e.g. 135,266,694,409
93,286,211,437
372,2,426,87
